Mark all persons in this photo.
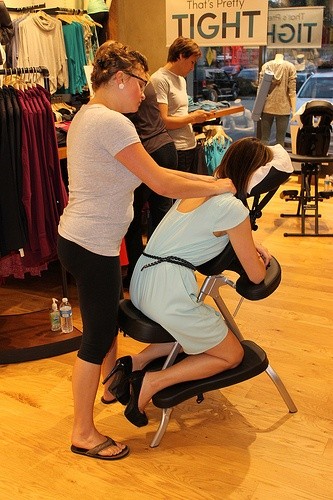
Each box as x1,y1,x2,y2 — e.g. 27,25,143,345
256,54,296,146
103,136,291,428
58,35,237,461
293,54,320,98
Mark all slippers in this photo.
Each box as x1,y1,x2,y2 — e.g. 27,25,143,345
71,436,129,460
101,395,117,403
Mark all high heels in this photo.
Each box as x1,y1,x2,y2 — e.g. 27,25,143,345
111,369,148,427
102,355,132,405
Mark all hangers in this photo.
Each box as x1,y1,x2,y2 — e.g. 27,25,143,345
193,124,232,146
0,4,104,92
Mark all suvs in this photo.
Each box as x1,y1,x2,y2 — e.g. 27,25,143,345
199,68,240,103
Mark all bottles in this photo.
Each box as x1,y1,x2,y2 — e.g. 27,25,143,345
59,298,73,333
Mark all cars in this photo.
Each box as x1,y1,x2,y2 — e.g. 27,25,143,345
295,72,314,92
286,72,333,140
223,65,238,78
235,68,259,95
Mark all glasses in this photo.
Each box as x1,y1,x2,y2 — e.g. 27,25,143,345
124,72,148,92
